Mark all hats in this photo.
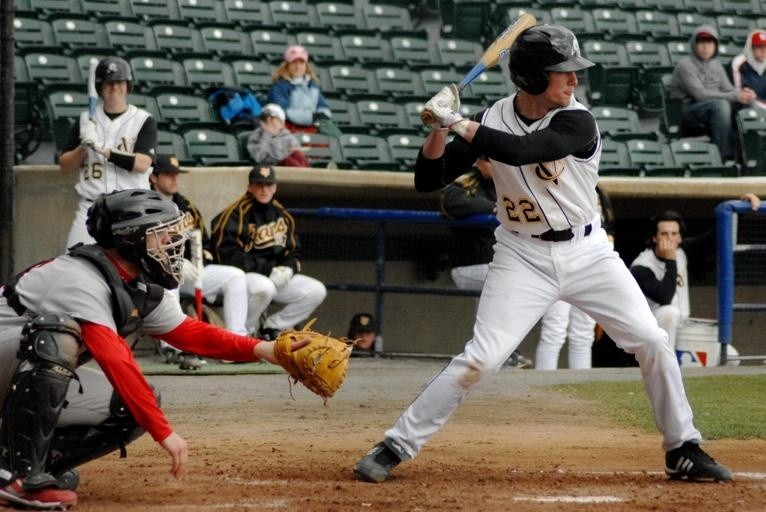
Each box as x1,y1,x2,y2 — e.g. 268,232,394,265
260,103,286,121
352,313,376,332
285,45,309,63
510,23,596,73
752,32,766,46
249,165,276,184
152,154,189,173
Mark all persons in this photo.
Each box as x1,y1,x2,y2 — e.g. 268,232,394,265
669,24,755,167
268,45,332,158
337,311,375,355
534,176,617,371
53,55,159,257
0,185,353,511
438,152,535,369
630,207,692,355
147,154,248,368
210,164,327,343
247,102,312,167
354,21,762,489
723,26,765,105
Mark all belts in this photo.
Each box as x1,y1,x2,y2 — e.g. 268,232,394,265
531,224,592,242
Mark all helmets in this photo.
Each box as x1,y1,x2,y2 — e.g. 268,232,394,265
95,57,134,97
86,188,187,290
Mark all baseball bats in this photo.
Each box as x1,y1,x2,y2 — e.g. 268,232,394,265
88,55,99,147
419,12,537,125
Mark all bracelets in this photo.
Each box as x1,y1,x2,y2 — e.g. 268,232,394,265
105,147,137,170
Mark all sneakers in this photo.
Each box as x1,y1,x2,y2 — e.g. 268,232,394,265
165,351,202,369
355,437,411,483
665,440,732,483
0,475,78,507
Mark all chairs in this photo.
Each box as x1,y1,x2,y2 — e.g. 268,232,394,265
13,0,766,178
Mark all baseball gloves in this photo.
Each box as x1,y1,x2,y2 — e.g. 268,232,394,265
275,327,355,397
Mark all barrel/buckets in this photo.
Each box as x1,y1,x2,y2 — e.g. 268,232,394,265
675,317,721,369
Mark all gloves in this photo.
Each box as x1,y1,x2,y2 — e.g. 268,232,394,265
79,134,106,154
425,83,461,129
